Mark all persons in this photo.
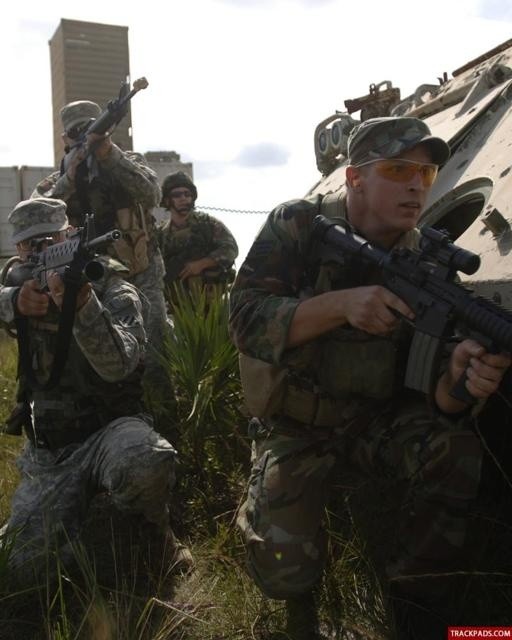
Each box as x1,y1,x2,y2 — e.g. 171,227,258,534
0,197,194,620
227,114,512,639
155,172,238,300
28,98,182,442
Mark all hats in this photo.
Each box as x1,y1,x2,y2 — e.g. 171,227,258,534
347,116,451,172
7,197,69,244
59,100,102,131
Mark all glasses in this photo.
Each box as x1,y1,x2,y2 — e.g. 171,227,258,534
356,157,440,186
19,231,59,250
67,128,85,139
171,191,193,198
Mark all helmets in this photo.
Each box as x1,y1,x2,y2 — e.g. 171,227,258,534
160,171,197,208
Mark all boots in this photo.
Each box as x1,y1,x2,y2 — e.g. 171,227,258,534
144,504,194,571
287,592,320,640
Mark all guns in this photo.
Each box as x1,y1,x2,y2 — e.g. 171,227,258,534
59,76,149,173
311,214,512,404
3,213,122,319
